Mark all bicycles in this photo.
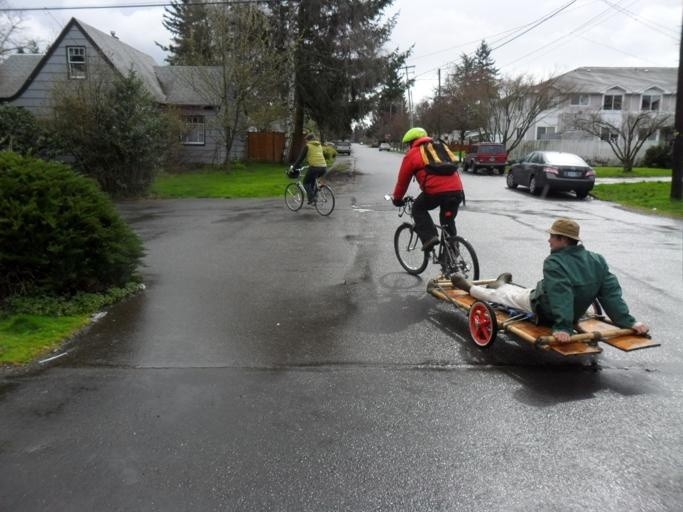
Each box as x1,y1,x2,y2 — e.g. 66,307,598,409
394,196,479,280
284,165,335,216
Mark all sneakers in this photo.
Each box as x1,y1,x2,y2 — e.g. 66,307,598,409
449,273,471,294
307,198,316,204
419,235,440,253
487,271,513,289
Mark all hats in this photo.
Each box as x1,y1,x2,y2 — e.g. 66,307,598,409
545,218,583,243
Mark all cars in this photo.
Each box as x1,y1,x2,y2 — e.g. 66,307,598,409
507,151,595,198
336,141,351,156
379,143,390,151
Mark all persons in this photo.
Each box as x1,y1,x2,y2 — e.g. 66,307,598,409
286,130,327,206
390,125,471,277
449,217,649,346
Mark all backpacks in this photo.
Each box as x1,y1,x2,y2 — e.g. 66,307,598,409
415,139,460,175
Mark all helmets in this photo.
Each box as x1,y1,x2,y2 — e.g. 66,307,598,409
401,127,427,145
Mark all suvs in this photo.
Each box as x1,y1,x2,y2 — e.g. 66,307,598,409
462,142,508,174
